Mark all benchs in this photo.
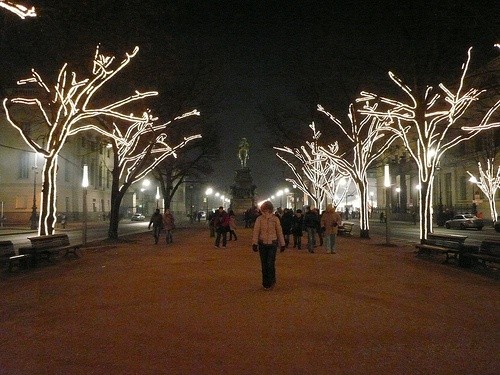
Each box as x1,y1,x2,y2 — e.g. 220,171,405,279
28,234,82,260
464,239,500,268
415,234,468,264
339,222,354,236
0,241,31,275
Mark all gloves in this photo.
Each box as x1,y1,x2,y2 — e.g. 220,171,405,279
279,246,286,252
252,244,258,252
321,227,325,232
333,222,338,227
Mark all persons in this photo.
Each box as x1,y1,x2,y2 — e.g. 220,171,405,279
320,206,342,254
345,209,350,220
276,206,284,223
61,214,67,229
305,205,320,254
293,210,304,249
478,211,483,218
282,208,293,248
207,209,219,238
102,212,109,220
213,206,229,249
193,210,202,222
410,212,417,225
163,211,175,245
252,201,286,290
379,212,384,223
149,209,164,245
244,207,262,229
320,211,326,247
227,209,238,241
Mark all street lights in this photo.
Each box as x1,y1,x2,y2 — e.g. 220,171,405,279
293,184,297,213
205,188,212,219
215,192,230,212
396,187,400,221
155,194,160,209
81,164,89,246
469,177,477,215
416,185,420,205
141,180,150,214
271,188,290,208
371,192,374,217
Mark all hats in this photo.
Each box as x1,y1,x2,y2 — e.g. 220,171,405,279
261,200,273,212
326,204,332,210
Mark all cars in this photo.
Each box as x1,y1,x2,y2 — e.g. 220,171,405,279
131,213,146,222
494,216,500,232
445,213,485,230
